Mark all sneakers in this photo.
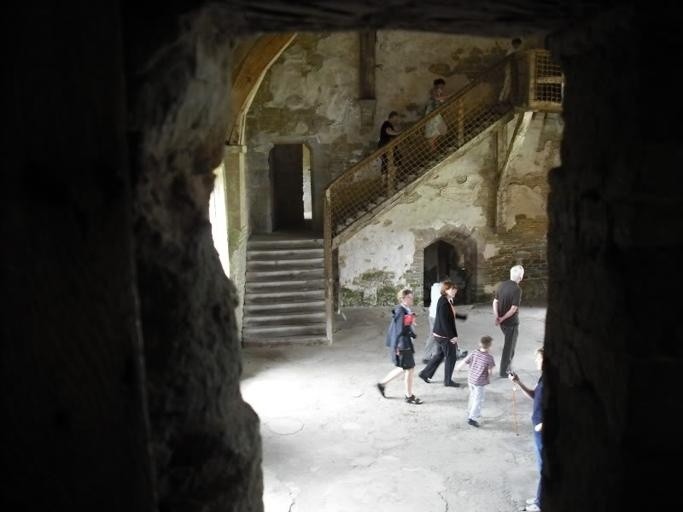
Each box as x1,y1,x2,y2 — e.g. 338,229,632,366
468,419,479,427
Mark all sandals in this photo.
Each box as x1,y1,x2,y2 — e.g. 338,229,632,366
404,394,423,404
378,384,384,396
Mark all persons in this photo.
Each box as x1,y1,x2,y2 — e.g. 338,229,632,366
424,78,448,158
457,336,496,427
376,288,424,405
418,282,460,387
378,110,404,188
497,37,522,105
492,265,524,378
508,348,543,512
422,273,468,364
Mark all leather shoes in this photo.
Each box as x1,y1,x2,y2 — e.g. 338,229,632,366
445,381,460,387
419,373,430,383
